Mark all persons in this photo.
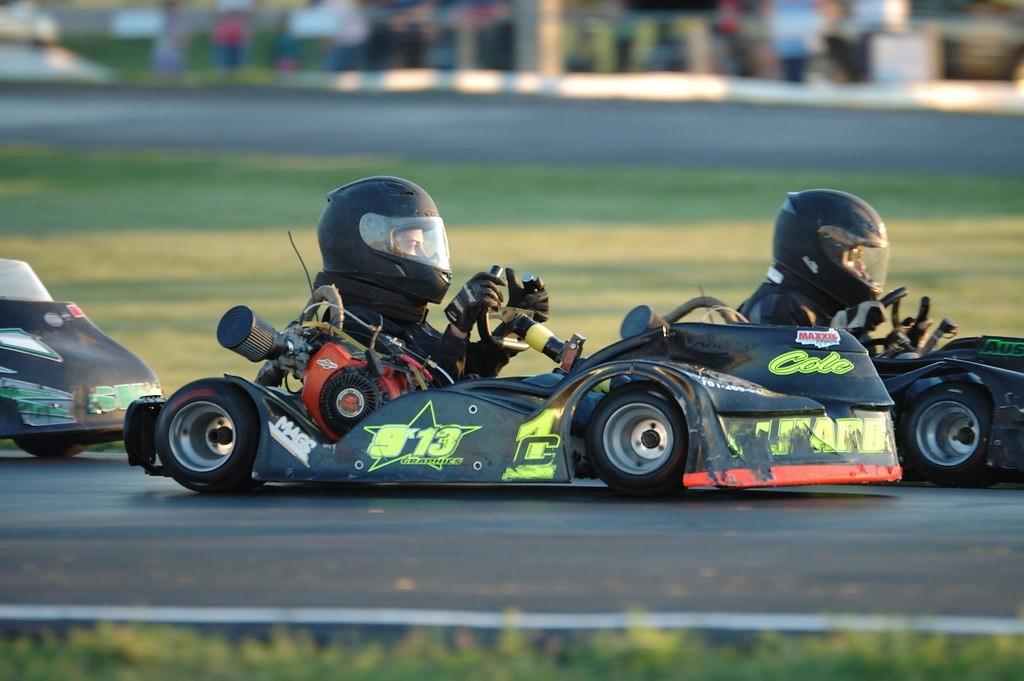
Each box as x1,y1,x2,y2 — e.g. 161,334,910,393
254,175,548,389
736,188,925,359
0,0,761,80
767,0,911,84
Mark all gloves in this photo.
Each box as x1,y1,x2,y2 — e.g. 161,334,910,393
444,272,506,332
830,301,886,341
885,317,932,350
492,269,549,337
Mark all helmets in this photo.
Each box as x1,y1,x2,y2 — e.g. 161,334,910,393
317,177,452,304
772,189,888,307
